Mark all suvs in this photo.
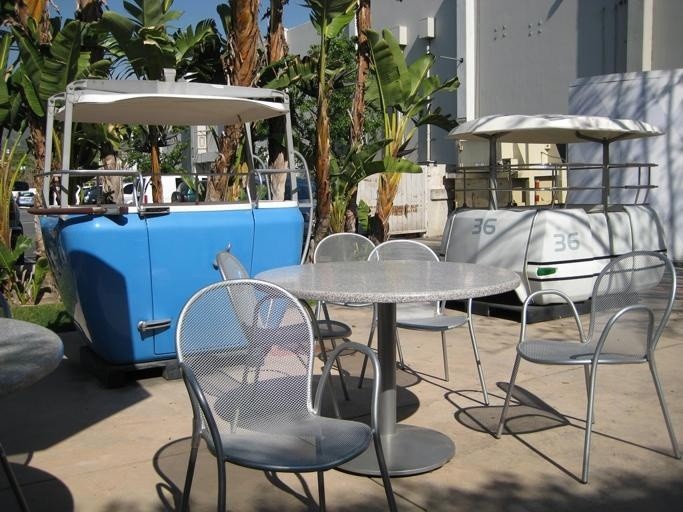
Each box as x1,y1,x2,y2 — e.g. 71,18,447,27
171,180,208,203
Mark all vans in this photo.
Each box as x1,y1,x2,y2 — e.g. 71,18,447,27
135,175,208,203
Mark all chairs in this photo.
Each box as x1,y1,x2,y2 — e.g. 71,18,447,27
310,232,393,379
174,278,400,512
494,248,681,484
216,250,355,435
368,240,452,393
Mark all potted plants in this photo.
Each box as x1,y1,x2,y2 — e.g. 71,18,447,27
1,235,64,330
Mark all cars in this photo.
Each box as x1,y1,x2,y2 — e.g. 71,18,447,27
16,191,36,207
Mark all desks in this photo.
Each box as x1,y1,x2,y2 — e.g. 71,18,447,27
1,316,67,511
250,258,522,479
